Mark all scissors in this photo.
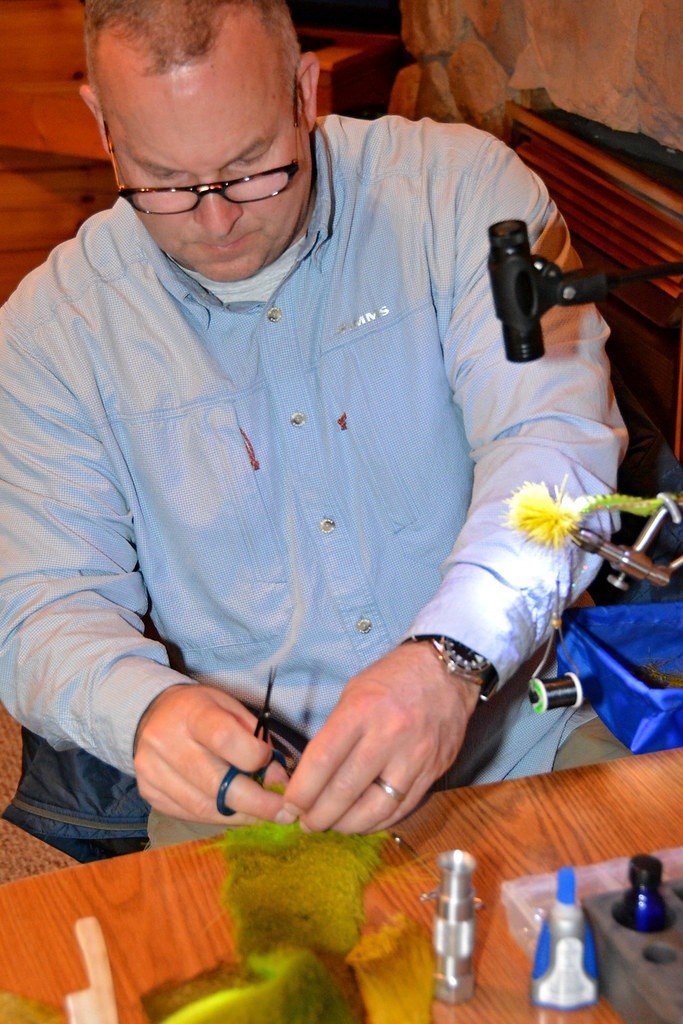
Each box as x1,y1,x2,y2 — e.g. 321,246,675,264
217,666,287,816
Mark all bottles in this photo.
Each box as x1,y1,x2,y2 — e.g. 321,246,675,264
527,869,600,1009
620,856,668,932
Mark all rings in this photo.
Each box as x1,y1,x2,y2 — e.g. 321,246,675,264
374,776,405,800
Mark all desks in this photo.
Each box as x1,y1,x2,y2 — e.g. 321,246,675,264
0,748,683,1024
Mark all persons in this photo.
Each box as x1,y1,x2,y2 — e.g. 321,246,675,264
0,1,635,836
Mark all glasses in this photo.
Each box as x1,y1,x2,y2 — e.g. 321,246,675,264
103,58,298,215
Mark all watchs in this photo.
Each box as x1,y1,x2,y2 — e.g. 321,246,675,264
402,635,498,702
